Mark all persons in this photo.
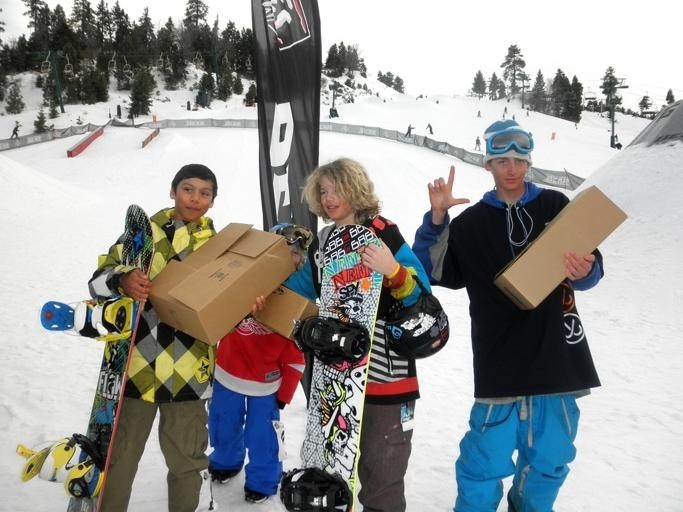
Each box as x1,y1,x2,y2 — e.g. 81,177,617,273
426,123,433,135
88,163,266,512
475,136,481,151
10,125,20,139
282,157,433,512
404,124,415,138
412,120,603,512
204,223,313,502
477,110,481,117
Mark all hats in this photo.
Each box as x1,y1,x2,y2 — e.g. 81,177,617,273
480,119,536,165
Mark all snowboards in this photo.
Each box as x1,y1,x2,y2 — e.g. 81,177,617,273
14,204,155,511
280,225,387,512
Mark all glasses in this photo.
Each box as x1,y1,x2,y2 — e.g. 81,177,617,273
276,225,314,249
486,127,534,154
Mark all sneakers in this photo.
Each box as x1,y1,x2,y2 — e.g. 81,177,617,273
244,491,270,504
210,462,243,482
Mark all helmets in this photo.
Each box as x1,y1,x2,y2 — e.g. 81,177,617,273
384,290,450,360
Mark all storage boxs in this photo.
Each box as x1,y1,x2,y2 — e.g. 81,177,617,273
493,185,627,310
149,223,296,347
249,285,319,341
